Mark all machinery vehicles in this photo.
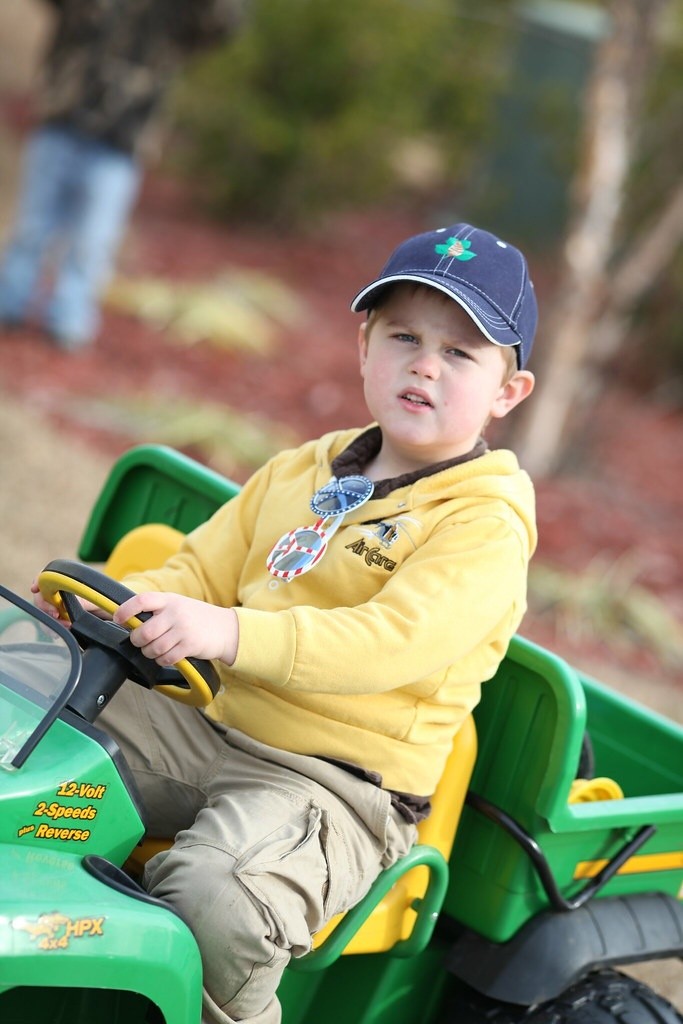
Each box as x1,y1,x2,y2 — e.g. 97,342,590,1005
0,443,683,1024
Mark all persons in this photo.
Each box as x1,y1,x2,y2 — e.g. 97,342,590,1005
0,1,246,353
0,225,541,1024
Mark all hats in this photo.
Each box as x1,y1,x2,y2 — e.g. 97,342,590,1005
350,224,538,372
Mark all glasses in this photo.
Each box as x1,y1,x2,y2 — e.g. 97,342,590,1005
266,474,374,583
